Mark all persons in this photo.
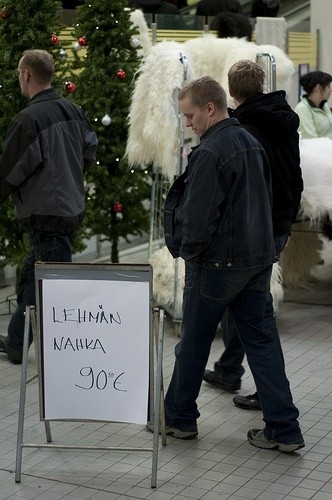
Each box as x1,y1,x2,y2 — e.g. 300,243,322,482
198,59,302,410
142,76,306,453
2,49,85,368
294,70,332,241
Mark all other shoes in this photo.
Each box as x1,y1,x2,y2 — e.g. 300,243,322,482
202,367,242,391
233,392,263,409
0,335,24,365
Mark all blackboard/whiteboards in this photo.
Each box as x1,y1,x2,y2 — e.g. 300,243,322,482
32,259,156,428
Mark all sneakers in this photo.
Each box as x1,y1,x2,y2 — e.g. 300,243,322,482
247,428,305,452
146,420,198,439
